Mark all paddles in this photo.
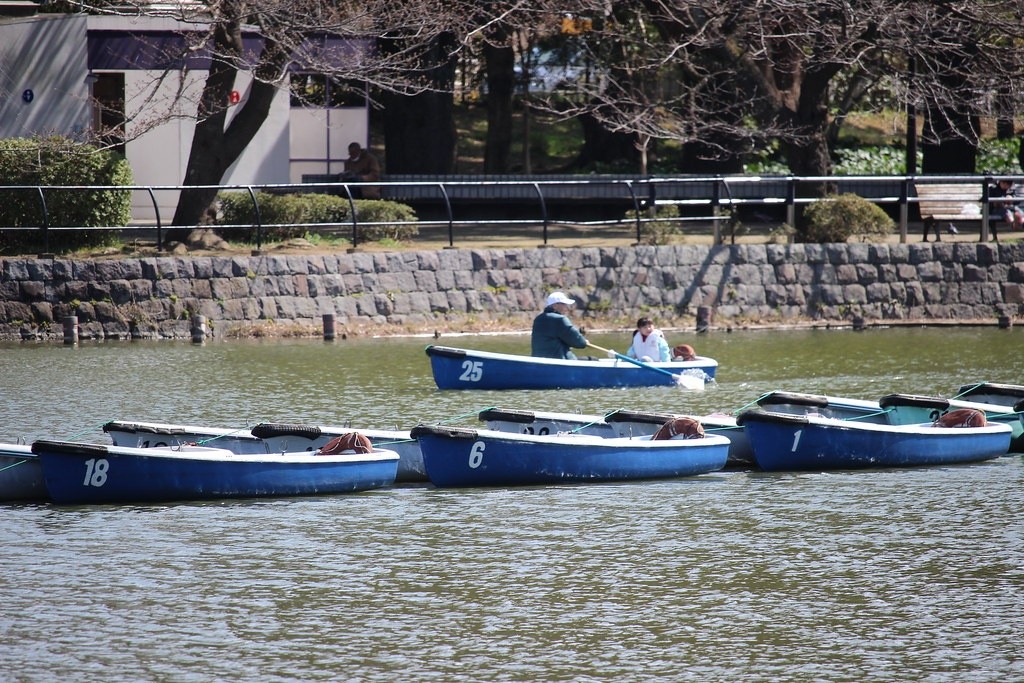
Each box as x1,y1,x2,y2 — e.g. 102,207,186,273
585,341,705,390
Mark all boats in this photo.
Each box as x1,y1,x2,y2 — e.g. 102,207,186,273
34,439,398,501
103,420,427,481
959,381,1023,408
603,408,747,458
877,394,1024,451
733,406,1014,471
479,408,612,440
428,345,720,392
0,441,36,508
407,422,731,485
755,391,889,425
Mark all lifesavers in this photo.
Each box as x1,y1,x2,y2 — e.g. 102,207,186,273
931,408,987,428
649,417,705,440
313,432,372,455
670,344,696,361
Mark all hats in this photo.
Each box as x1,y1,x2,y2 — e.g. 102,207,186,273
545,292,575,307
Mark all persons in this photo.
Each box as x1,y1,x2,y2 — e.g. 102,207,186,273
530,291,599,361
338,142,380,200
989,170,1024,231
626,316,671,362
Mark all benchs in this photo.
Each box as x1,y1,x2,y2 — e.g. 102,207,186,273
916,181,1024,241
